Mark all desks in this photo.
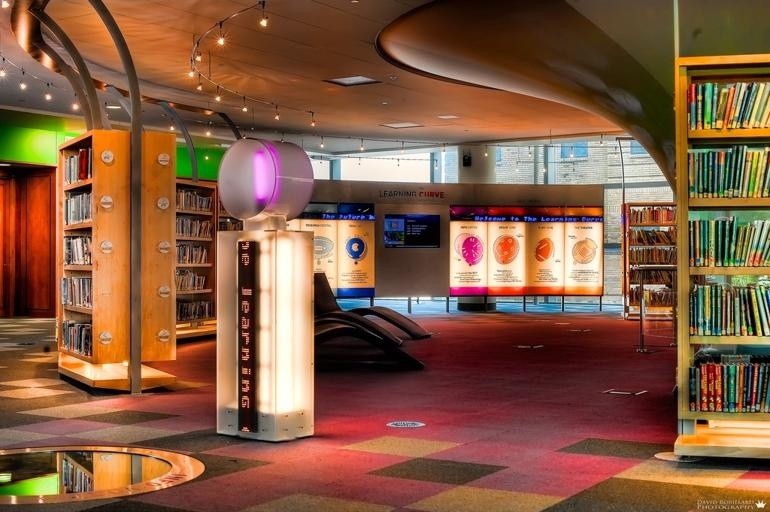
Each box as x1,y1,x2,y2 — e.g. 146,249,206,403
631,265,677,353
0,446,205,511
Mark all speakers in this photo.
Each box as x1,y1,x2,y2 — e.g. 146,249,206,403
463,154,471,167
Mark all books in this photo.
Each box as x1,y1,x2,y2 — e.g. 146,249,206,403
56,450,94,496
626,204,677,307
687,75,770,416
60,145,94,357
175,186,242,323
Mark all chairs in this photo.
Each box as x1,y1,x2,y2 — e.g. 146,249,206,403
314,273,431,372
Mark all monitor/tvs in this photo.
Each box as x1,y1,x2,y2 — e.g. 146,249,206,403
338,201,376,220
383,214,441,249
298,201,338,220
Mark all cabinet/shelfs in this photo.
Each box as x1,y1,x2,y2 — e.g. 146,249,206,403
56,129,177,394
675,54,770,461
176,178,217,339
621,202,677,307
217,181,243,231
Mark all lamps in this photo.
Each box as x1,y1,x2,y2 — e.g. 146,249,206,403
215,34,227,48
241,104,248,113
256,15,271,30
196,83,203,91
191,52,206,63
213,94,222,101
188,70,197,79
308,120,318,128
273,113,280,121
0,0,79,111
